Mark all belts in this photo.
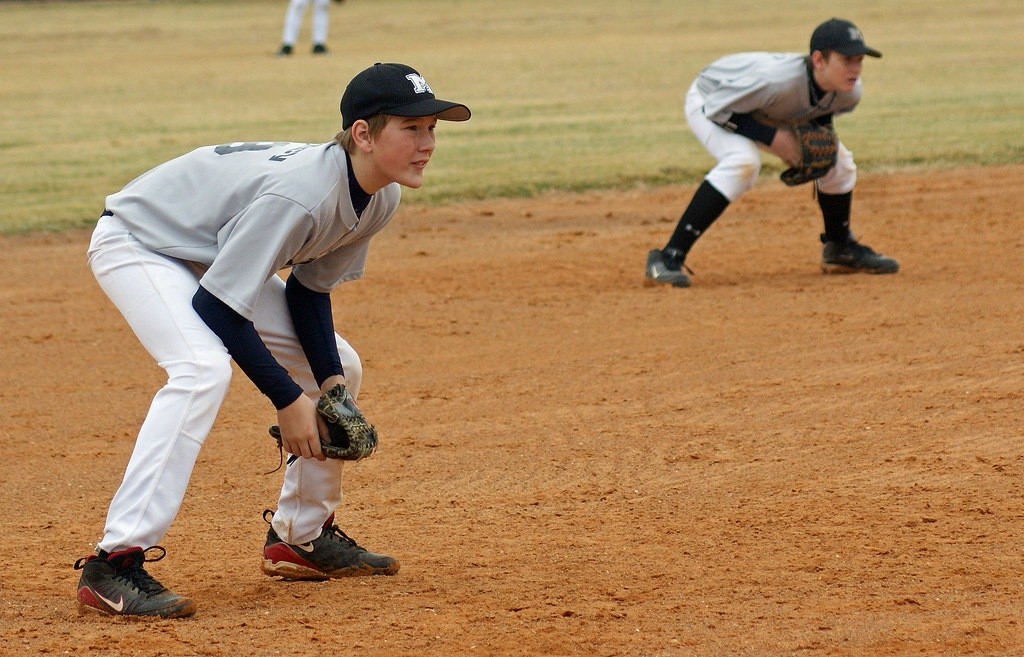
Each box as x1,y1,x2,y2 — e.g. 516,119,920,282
100,209,114,217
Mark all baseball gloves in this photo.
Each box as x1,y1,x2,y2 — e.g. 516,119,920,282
269,384,378,461
779,126,838,185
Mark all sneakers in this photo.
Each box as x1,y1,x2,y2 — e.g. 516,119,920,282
261,508,400,580
73,545,197,617
644,248,694,287
823,238,899,274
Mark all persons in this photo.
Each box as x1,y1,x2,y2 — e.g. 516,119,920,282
642,17,899,288
73,63,472,621
268,0,330,57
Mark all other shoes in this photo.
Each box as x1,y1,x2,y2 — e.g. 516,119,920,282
282,46,290,55
313,45,324,52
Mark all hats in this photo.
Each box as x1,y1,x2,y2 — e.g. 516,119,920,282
810,19,882,59
340,63,471,132
